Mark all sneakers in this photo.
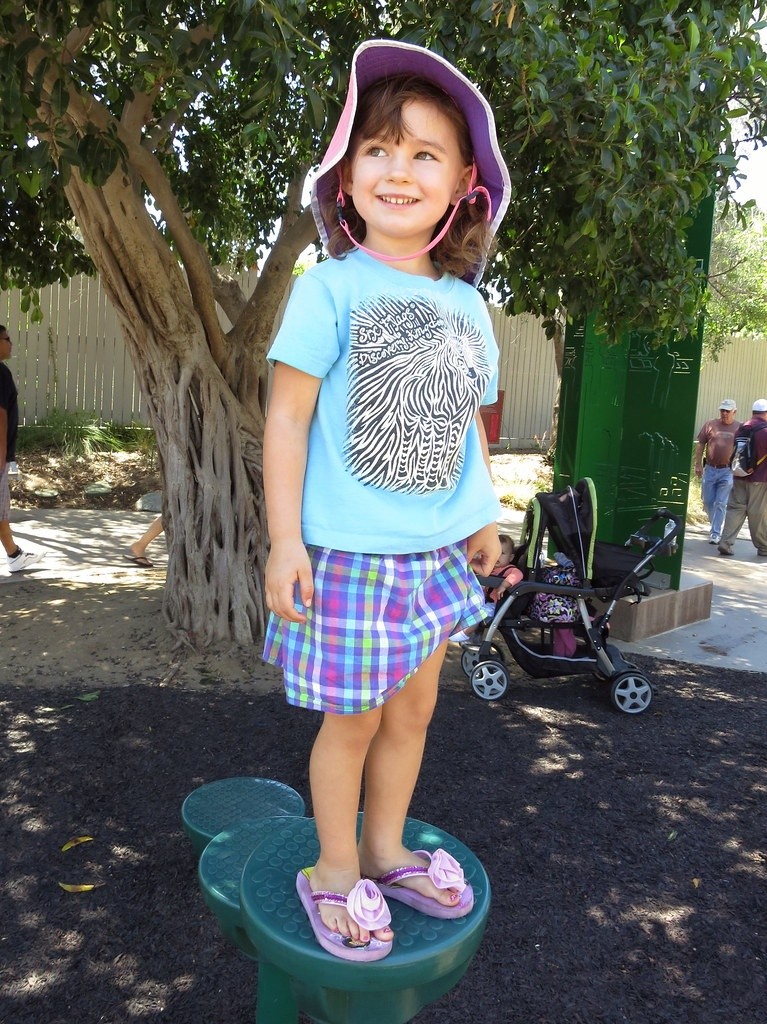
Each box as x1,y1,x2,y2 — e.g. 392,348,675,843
710,532,719,544
8,548,48,573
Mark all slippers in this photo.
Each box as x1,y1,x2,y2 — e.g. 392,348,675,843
123,552,152,567
360,849,474,919
295,866,394,962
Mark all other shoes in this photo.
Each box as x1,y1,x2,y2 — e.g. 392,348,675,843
718,542,734,555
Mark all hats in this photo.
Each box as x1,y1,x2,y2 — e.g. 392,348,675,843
752,399,767,411
306,38,513,293
719,399,736,412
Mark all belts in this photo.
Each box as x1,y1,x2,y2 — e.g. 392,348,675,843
706,462,729,469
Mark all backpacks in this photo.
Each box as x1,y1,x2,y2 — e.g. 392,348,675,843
730,424,767,476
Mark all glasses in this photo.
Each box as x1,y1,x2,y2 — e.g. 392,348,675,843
0,337,10,342
720,408,730,413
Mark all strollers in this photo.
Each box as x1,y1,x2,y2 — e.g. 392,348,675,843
459,477,682,714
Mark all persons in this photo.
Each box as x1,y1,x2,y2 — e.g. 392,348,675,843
262,39,513,961
0,326,47,573
123,514,164,568
696,399,742,545
463,534,523,635
718,399,767,556
